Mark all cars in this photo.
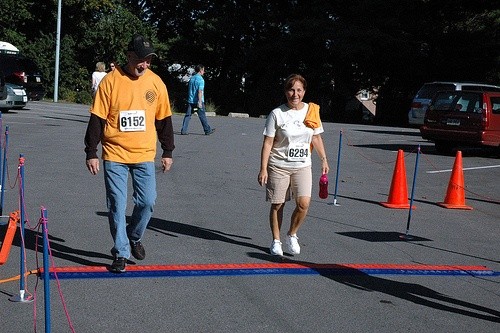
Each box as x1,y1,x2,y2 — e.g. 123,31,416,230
419,90,500,156
407,81,500,128
0,81,28,114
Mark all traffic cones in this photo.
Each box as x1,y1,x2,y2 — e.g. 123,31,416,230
377,149,418,210
435,150,475,210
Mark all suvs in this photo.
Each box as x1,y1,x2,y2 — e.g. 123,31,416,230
0,41,48,102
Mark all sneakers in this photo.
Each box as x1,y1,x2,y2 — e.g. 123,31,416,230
270,240,284,256
110,257,127,272
205,128,216,135
129,239,146,260
286,233,300,255
181,132,189,135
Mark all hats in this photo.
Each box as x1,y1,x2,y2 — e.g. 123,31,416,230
128,35,158,59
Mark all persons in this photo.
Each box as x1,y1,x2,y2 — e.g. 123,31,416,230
84,35,175,272
92,62,107,96
181,65,215,136
106,61,118,74
258,75,329,255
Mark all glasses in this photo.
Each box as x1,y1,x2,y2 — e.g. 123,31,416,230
109,65,115,67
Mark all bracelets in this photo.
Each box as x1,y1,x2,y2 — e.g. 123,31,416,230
320,156,327,161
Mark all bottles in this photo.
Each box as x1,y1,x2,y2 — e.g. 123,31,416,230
193,108,203,111
319,172,329,199
